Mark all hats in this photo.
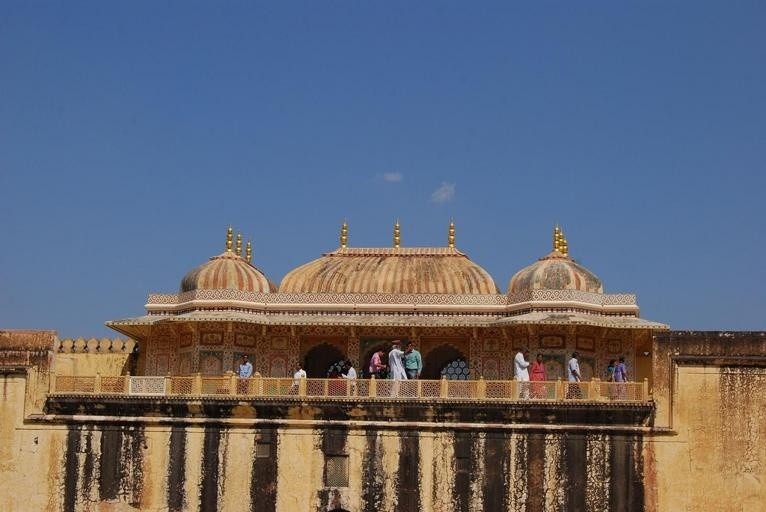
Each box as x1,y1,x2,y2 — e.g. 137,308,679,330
391,339,401,344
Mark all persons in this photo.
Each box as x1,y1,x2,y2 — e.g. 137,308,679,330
565,351,583,399
404,343,423,378
616,355,630,402
511,348,548,399
368,349,386,379
290,362,307,394
237,354,253,395
342,361,357,395
606,359,617,400
390,342,414,398
